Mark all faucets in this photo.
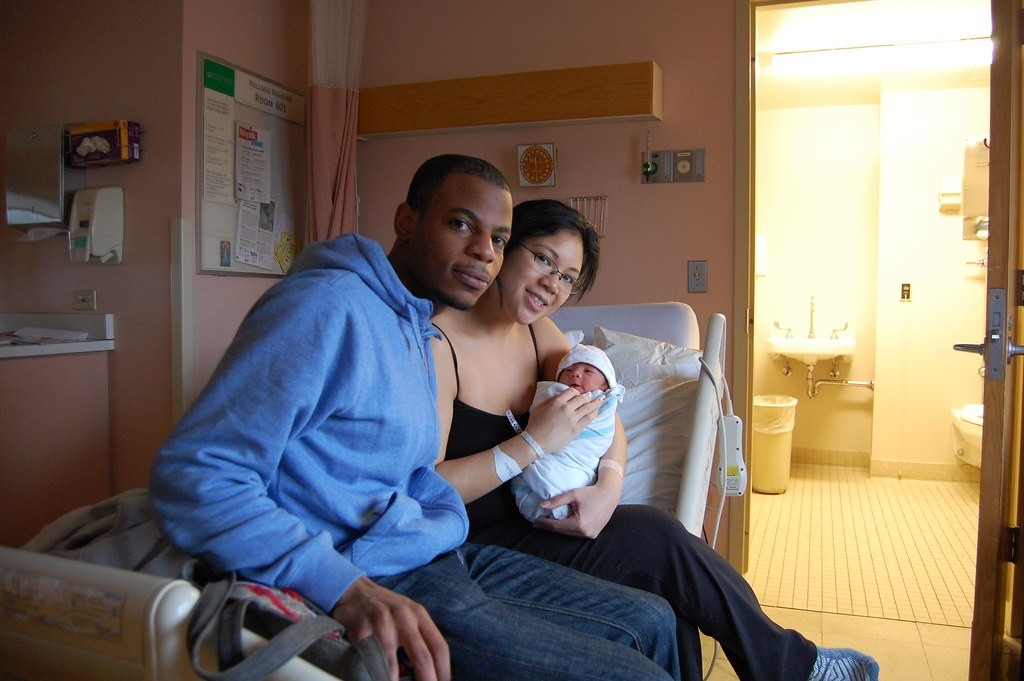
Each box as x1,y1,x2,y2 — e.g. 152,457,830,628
809,296,816,339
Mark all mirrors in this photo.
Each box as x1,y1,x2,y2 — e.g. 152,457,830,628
963,144,990,240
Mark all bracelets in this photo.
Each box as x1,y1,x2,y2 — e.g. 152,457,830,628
598,459,624,477
492,446,522,482
505,410,544,457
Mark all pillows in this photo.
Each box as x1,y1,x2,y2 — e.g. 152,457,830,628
592,324,703,388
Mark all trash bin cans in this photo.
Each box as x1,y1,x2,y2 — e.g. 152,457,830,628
751,395,799,495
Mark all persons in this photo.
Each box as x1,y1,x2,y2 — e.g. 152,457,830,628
511,344,625,527
154,154,682,681
426,198,880,681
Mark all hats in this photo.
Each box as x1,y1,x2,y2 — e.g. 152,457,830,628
556,343,617,388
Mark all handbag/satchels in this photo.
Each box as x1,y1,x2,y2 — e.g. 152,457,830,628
186,566,387,680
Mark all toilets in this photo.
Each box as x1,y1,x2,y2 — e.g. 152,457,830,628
952,404,984,469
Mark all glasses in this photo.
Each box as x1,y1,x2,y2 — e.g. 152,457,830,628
517,242,584,294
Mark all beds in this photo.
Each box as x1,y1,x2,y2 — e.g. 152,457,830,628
0,300,746,681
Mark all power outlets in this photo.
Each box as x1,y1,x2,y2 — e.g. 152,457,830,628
900,281,912,302
687,260,709,293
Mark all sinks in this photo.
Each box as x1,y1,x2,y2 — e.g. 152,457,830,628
766,337,856,366
